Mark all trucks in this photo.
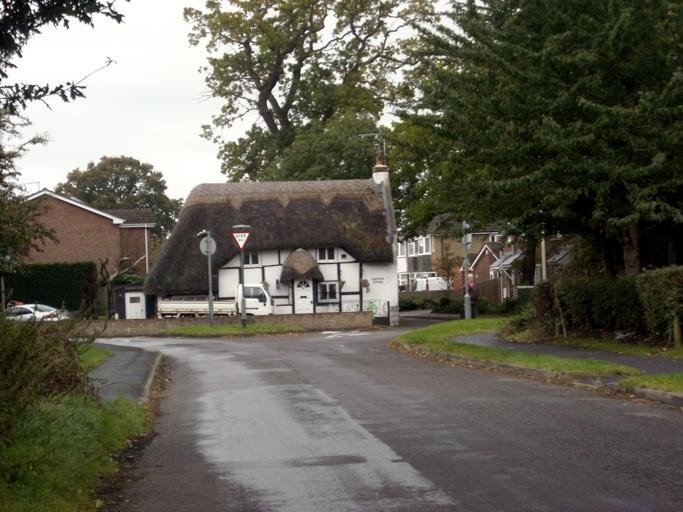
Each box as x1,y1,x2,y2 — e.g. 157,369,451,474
155,282,274,319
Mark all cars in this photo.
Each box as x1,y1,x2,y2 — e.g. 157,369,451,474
0,302,69,323
